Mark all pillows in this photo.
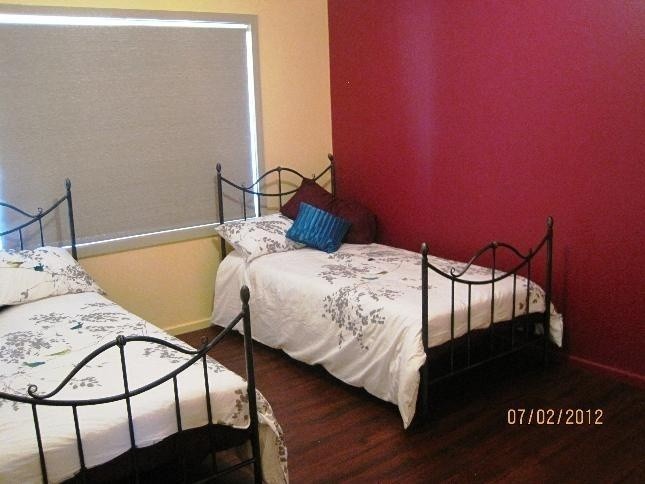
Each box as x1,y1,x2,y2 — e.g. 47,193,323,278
279,177,338,221
0,245,107,308
214,211,307,263
284,200,352,253
337,203,378,245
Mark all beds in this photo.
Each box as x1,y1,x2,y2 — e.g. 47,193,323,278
208,152,565,430
0,177,290,483
